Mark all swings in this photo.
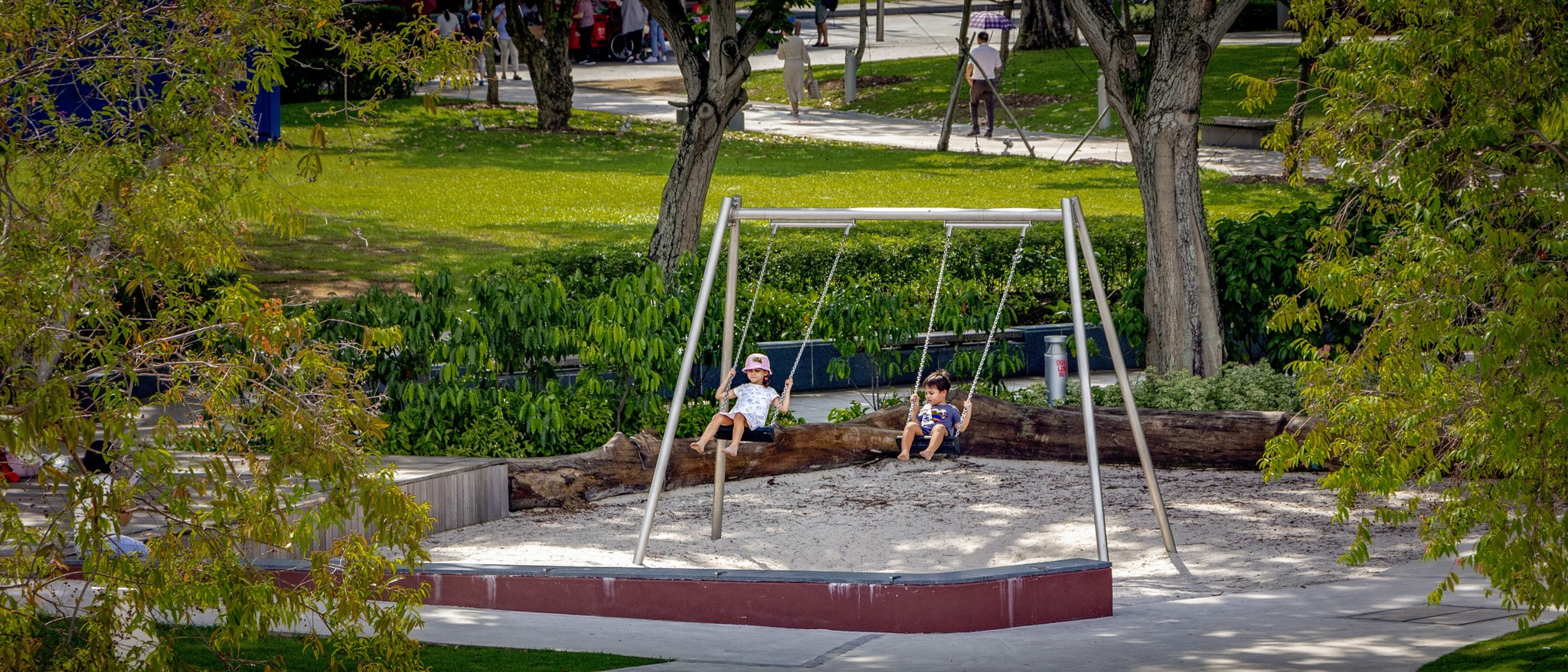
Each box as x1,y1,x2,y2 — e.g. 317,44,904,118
895,221,1030,455
714,222,855,443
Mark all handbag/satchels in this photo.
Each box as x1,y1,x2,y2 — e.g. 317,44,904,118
803,69,813,89
806,67,821,99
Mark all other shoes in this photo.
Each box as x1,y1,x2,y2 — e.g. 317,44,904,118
466,80,473,85
644,55,657,63
502,75,506,79
514,75,522,80
967,129,981,136
984,130,993,137
788,110,799,115
626,55,634,63
812,42,821,47
579,59,588,63
818,42,829,47
585,60,596,65
480,81,484,85
634,58,643,63
658,55,667,61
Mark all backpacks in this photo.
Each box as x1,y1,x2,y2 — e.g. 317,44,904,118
822,0,838,11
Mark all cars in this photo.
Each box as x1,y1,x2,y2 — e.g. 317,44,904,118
554,2,634,63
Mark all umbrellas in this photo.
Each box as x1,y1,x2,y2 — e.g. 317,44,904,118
953,11,1019,32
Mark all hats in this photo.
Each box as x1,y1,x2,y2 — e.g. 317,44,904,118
741,353,773,376
469,12,480,23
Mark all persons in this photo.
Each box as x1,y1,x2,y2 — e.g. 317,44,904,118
690,353,793,456
463,13,485,86
773,5,801,37
493,2,524,80
521,0,541,25
645,11,668,62
572,0,596,65
897,368,972,461
809,0,829,47
966,31,1002,138
432,3,461,84
619,0,649,64
777,21,811,116
460,0,487,80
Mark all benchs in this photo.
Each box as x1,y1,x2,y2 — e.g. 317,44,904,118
1200,116,1285,150
668,100,752,131
